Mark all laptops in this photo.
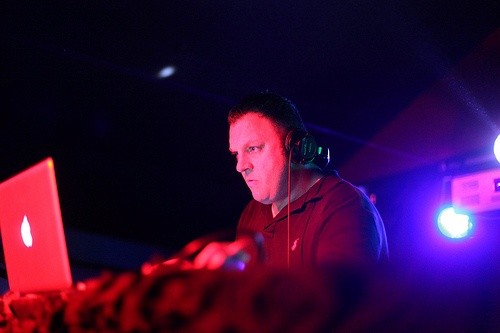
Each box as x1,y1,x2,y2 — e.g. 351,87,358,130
0,157,82,295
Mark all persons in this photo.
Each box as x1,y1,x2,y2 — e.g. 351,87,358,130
229,94,395,267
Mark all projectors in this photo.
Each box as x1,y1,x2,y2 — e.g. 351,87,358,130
450,166,500,218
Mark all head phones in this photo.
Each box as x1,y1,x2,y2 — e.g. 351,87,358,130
284,127,330,170
